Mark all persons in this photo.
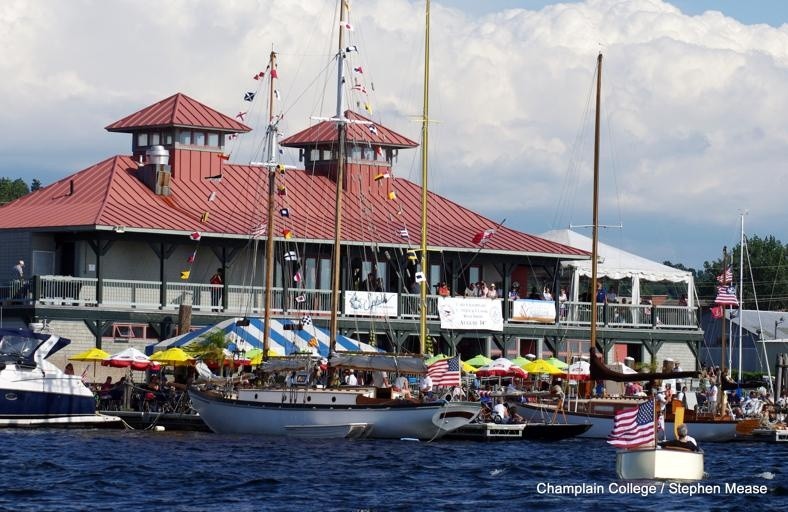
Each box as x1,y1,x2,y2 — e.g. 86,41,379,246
353,267,384,292
210,268,223,312
442,379,565,424
463,280,497,300
508,286,520,300
309,365,436,401
13,260,25,280
98,373,177,407
64,363,74,375
543,287,553,301
438,281,450,296
594,380,605,397
622,364,788,451
558,287,567,317
580,281,652,324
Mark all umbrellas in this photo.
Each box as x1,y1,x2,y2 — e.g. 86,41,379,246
69,345,197,381
426,352,590,387
209,347,277,368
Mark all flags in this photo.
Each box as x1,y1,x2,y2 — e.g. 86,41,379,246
716,266,740,306
606,400,655,450
471,226,495,245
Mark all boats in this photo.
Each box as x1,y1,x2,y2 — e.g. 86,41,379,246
612,388,706,483
